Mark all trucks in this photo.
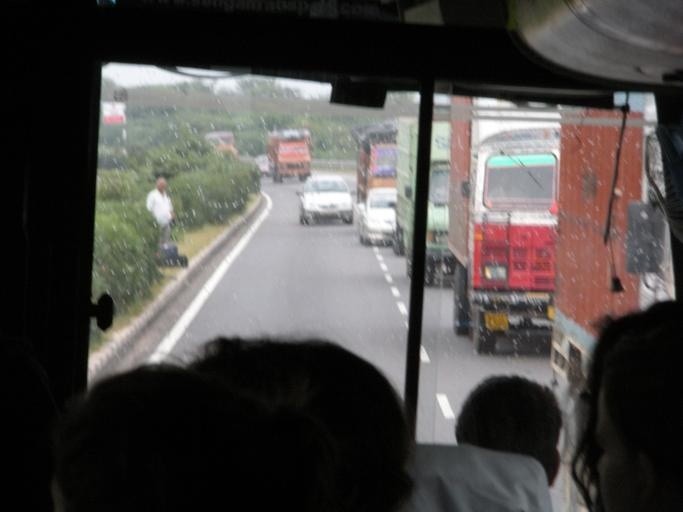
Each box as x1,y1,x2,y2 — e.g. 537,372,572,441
205,130,238,159
267,128,311,183
357,131,396,196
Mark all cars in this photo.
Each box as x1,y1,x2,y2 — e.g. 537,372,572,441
356,188,397,246
298,173,356,225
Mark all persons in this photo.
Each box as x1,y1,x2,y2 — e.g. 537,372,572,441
60,301,683,512
146,177,174,261
